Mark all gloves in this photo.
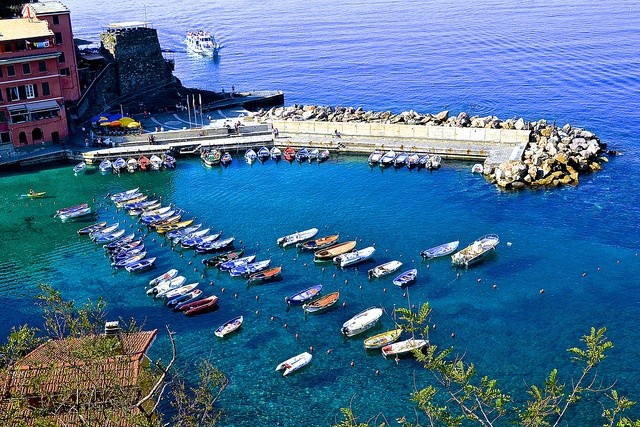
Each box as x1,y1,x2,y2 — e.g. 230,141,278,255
89,222,119,238
381,150,395,163
274,352,312,376
421,241,460,259
257,147,269,158
113,158,126,171
110,187,139,200
114,244,145,262
382,336,429,357
164,282,199,300
368,151,384,163
249,267,281,280
21,192,47,198
57,204,88,215
141,206,171,216
123,200,158,210
157,220,193,234
104,233,135,248
308,149,319,159
318,150,329,160
333,246,376,268
115,251,147,269
138,156,150,170
245,149,257,160
150,155,162,169
472,163,483,173
406,154,419,166
96,229,125,243
161,154,176,170
368,261,402,278
116,196,148,208
284,147,295,161
229,260,270,277
79,222,106,236
129,203,161,216
112,240,142,256
166,289,203,309
364,328,403,348
452,234,500,266
426,155,441,169
126,158,137,171
393,269,418,287
295,148,308,162
125,257,157,273
296,234,339,250
314,240,356,263
114,193,142,204
179,295,219,316
303,292,339,314
214,315,243,338
150,215,181,227
270,147,281,159
181,234,220,249
147,276,186,295
59,207,91,220
196,237,234,253
276,228,319,248
418,154,429,164
167,224,202,241
201,250,243,267
201,149,221,165
215,255,256,271
285,284,322,305
221,152,232,165
141,210,176,226
99,159,111,171
149,268,178,286
394,152,407,164
341,307,383,337
73,162,85,172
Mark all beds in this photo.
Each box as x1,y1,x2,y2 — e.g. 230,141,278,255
99,122,111,126
99,113,111,116
109,116,119,120
101,119,114,122
87,116,100,122
109,120,121,126
113,113,124,117
127,121,140,128
119,116,135,128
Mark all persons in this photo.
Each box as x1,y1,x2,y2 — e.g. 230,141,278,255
149,134,155,145
185,105,188,112
29,189,34,195
126,112,130,118
237,121,240,125
209,114,212,119
131,114,134,119
335,128,337,133
85,137,90,146
154,126,159,132
206,114,209,119
97,138,102,148
175,104,180,112
274,128,277,136
143,110,147,118
160,126,165,132
198,130,203,137
106,140,109,148
202,131,205,136
233,123,237,131
